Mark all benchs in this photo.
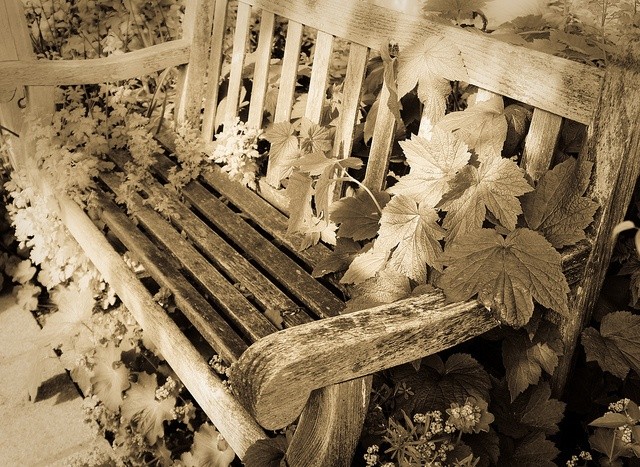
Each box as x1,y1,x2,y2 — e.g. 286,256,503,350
2,0,639,467
